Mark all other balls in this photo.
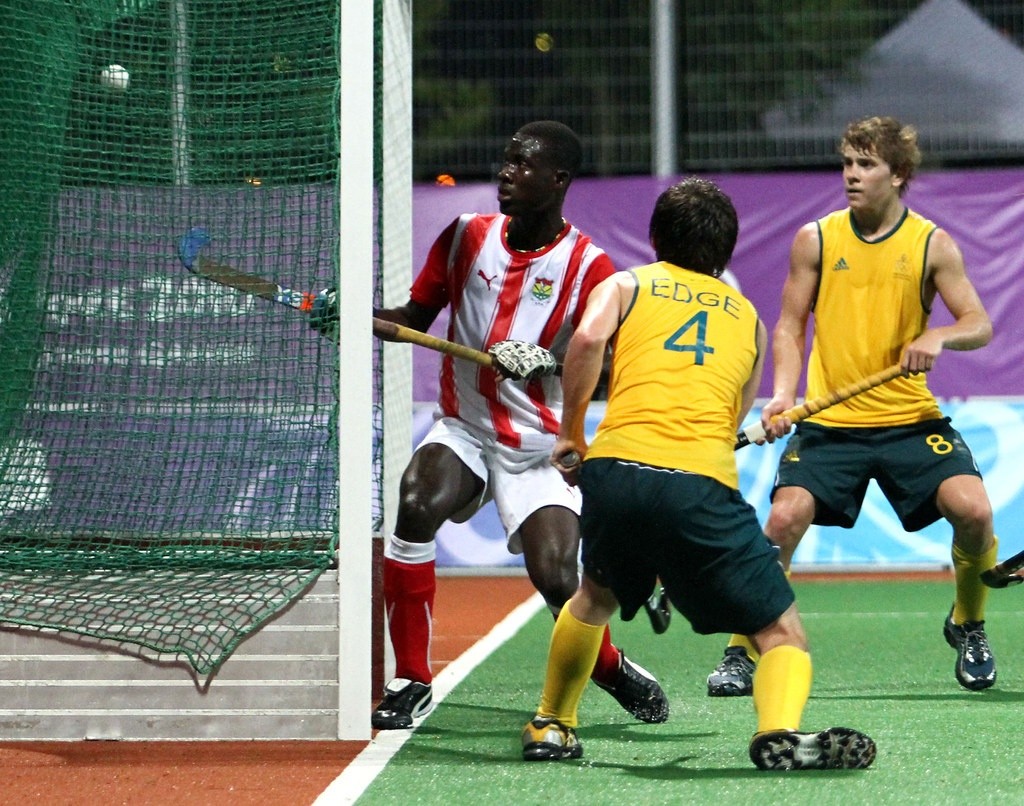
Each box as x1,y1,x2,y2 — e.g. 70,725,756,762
98,61,131,92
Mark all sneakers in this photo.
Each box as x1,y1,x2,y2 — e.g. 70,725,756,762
943,603,996,691
645,585,671,634
521,719,581,761
590,645,668,723
749,725,876,770
706,646,755,696
372,679,432,730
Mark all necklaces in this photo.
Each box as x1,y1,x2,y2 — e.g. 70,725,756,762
504,215,566,254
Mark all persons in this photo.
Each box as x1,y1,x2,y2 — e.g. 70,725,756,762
701,116,1001,697
310,116,673,728
519,175,880,772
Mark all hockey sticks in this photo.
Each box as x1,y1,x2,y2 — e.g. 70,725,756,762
560,447,676,639
727,360,911,456
975,550,1024,594
170,224,498,371
